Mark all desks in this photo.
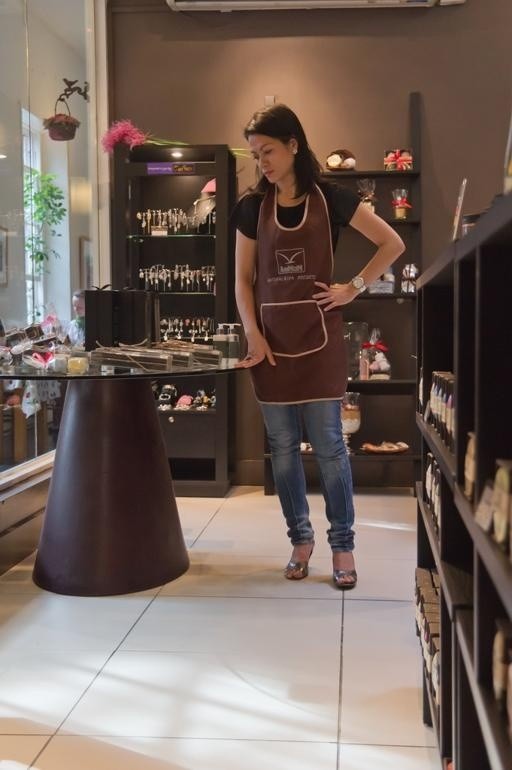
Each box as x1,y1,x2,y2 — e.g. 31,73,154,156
0,358,248,597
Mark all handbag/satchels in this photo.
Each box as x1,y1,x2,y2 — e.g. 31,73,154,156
84,290,161,351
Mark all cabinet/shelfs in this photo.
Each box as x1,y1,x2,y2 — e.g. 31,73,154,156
263,169,421,496
111,142,237,485
411,195,512,770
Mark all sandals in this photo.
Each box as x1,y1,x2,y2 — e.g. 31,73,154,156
332,552,357,589
283,542,314,580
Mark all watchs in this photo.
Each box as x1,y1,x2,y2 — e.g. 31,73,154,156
352,276,366,293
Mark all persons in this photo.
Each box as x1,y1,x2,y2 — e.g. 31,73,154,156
232,103,406,589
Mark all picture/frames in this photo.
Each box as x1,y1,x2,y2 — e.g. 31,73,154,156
0,225,8,288
78,234,91,289
